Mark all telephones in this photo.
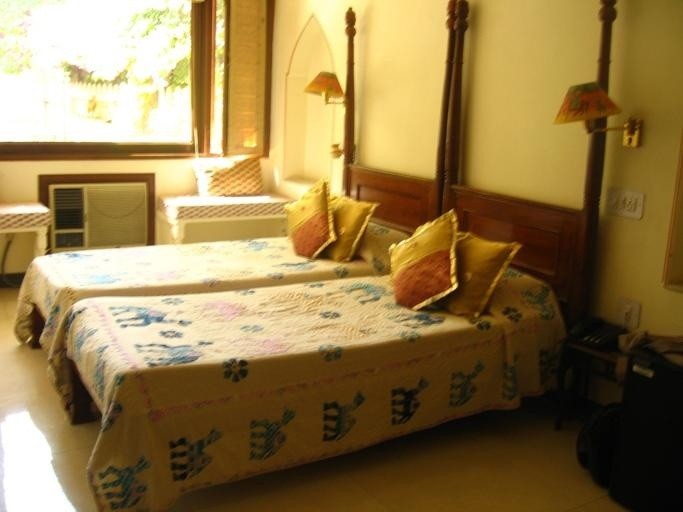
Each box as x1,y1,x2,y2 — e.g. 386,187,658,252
568,315,627,351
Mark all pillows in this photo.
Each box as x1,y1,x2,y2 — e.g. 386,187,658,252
191,154,264,197
283,180,551,318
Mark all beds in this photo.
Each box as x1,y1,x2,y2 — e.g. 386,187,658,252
46,1,616,512
13,1,455,350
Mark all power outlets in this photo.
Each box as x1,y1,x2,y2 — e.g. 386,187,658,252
619,301,641,328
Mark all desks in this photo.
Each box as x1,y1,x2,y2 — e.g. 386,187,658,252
553,340,616,431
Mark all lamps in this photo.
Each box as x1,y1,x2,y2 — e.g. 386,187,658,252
304,72,347,104
553,82,643,149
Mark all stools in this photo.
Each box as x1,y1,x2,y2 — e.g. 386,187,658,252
0,204,51,256
157,191,298,244
